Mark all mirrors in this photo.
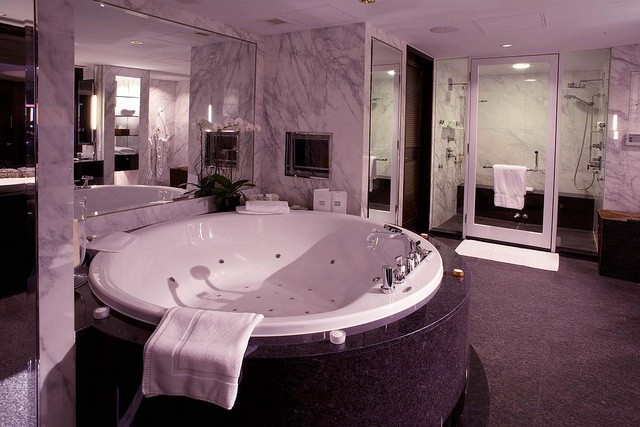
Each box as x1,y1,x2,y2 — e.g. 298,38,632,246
364,36,403,224
0,1,40,425
74,0,257,224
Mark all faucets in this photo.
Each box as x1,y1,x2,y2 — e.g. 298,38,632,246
367,232,417,270
587,161,601,171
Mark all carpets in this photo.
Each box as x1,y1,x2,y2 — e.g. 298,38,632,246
455,238,559,272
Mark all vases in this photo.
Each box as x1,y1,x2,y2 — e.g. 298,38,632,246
217,193,241,212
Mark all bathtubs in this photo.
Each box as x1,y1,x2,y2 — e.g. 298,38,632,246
73,183,187,220
90,208,443,327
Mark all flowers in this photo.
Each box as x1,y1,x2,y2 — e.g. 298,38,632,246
179,119,212,197
212,117,261,212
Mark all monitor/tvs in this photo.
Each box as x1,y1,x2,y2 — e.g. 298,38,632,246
214,135,235,160
293,138,331,173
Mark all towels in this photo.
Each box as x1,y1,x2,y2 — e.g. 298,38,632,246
141,305,265,413
245,201,291,215
493,164,529,211
